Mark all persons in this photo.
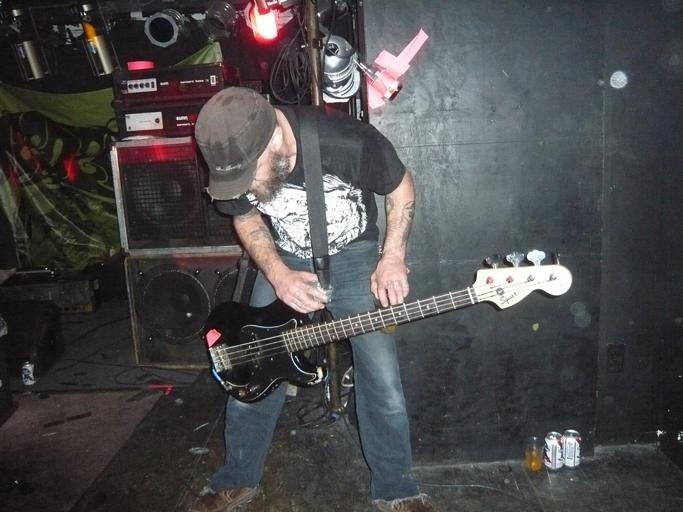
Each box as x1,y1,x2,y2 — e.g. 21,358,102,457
192,84,438,512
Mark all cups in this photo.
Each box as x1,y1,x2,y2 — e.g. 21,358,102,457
524,436,545,470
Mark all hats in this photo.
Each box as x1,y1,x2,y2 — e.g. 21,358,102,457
193,86,276,201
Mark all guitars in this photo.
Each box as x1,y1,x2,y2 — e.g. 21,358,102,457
204,249,573,404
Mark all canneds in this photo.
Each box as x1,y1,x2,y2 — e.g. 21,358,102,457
562,429,581,467
543,431,563,469
21,361,36,386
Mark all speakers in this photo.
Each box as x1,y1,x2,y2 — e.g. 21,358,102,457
109,136,261,256
119,253,243,368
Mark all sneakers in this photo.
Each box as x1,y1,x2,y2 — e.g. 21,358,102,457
373,493,436,512
188,482,259,511
